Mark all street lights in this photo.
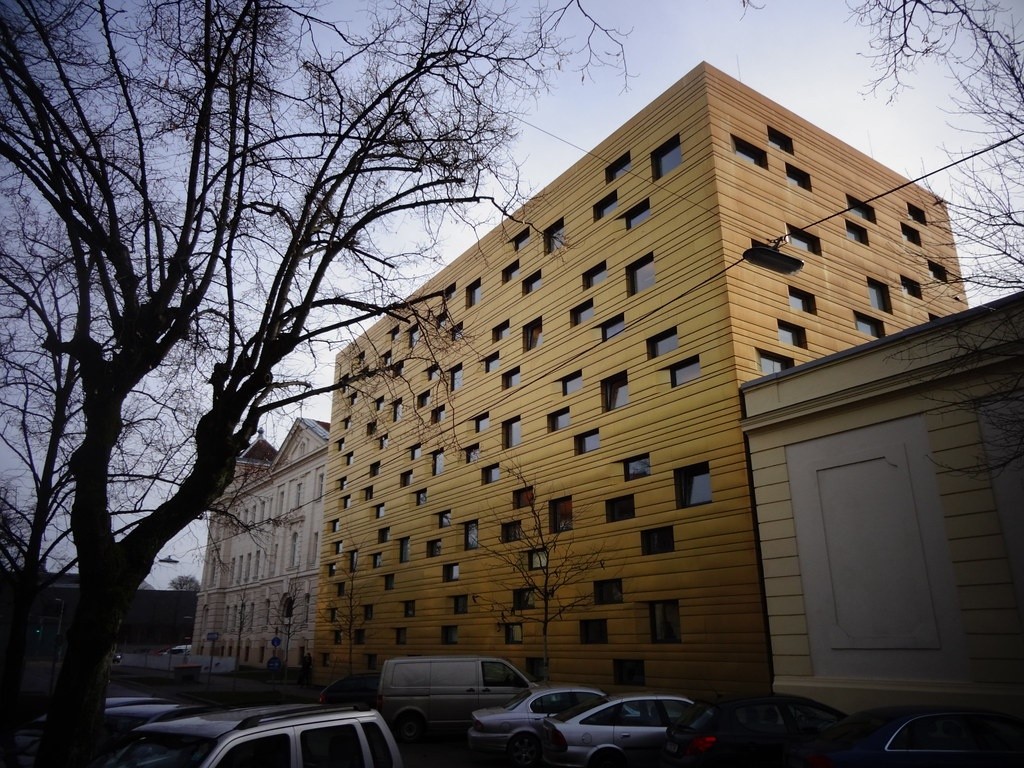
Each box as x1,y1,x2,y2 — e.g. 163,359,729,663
55,597,65,636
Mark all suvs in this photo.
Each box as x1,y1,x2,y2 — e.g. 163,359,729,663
91,701,403,768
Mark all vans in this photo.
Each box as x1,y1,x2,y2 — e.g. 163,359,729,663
377,656,550,743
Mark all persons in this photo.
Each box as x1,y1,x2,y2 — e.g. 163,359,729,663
297,652,312,688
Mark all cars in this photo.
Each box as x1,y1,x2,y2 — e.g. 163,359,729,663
113,648,124,663
162,645,191,655
466,684,619,768
659,692,850,768
14,697,169,764
544,694,696,768
104,704,225,749
794,704,1024,768
318,672,382,705
